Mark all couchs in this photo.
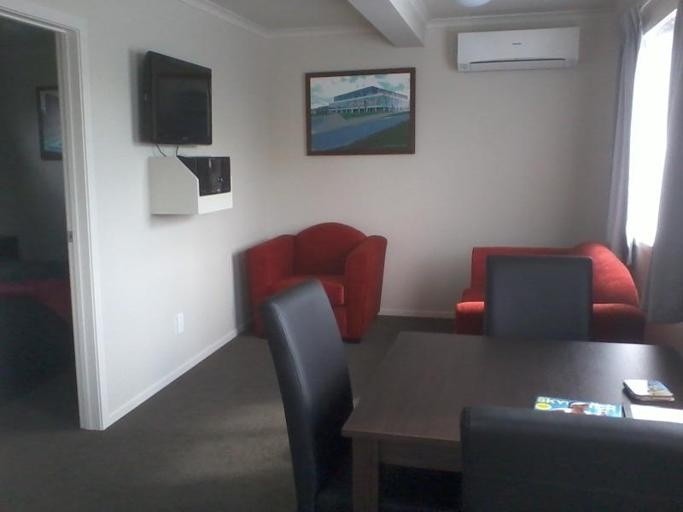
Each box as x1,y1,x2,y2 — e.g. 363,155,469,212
455,241,644,343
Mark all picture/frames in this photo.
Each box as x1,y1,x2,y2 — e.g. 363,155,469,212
304,66,415,156
35,86,61,161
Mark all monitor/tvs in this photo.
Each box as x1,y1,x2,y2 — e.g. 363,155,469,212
147,50,213,145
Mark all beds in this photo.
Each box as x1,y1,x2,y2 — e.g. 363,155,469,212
1,237,73,382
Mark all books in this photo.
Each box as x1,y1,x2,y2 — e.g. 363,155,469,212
534,379,683,423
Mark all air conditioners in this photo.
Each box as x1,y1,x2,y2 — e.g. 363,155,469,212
456,25,580,73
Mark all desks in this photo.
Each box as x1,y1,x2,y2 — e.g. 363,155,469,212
341,329,679,510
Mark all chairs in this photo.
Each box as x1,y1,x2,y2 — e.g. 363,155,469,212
244,224,387,342
458,403,680,512
261,277,355,508
480,255,592,344
